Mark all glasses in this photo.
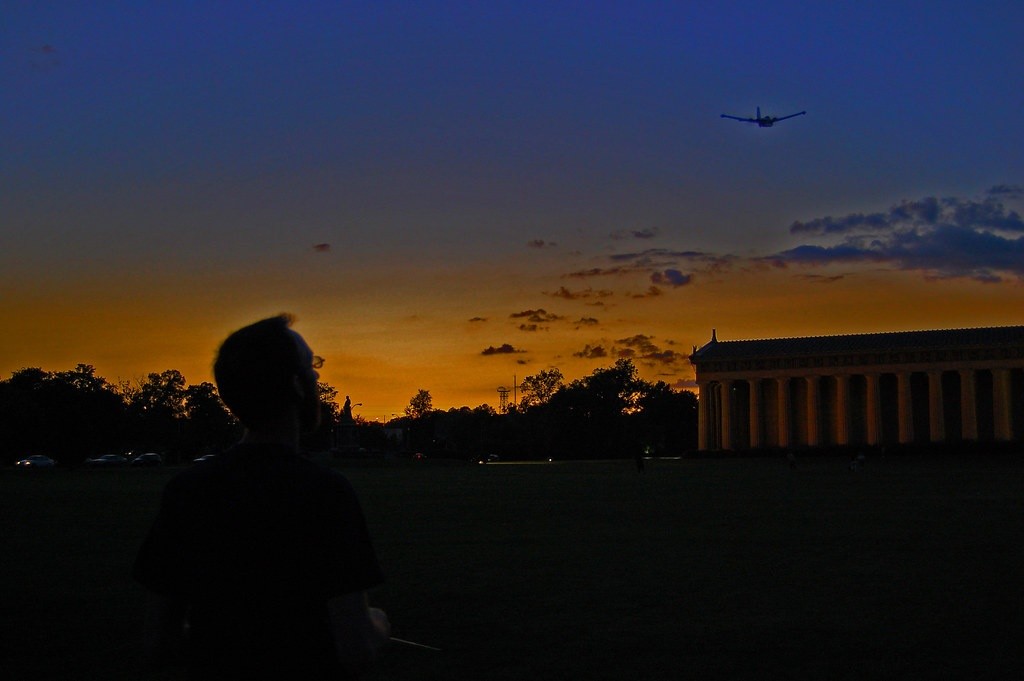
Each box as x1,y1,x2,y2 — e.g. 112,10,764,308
297,356,324,375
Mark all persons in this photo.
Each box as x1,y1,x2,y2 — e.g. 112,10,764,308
134,316,391,681
786,448,797,472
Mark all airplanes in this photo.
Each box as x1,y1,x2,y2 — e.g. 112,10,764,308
721,105,808,128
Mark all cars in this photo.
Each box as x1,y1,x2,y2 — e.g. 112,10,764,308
89,454,128,467
14,454,55,468
192,454,216,465
133,452,162,467
